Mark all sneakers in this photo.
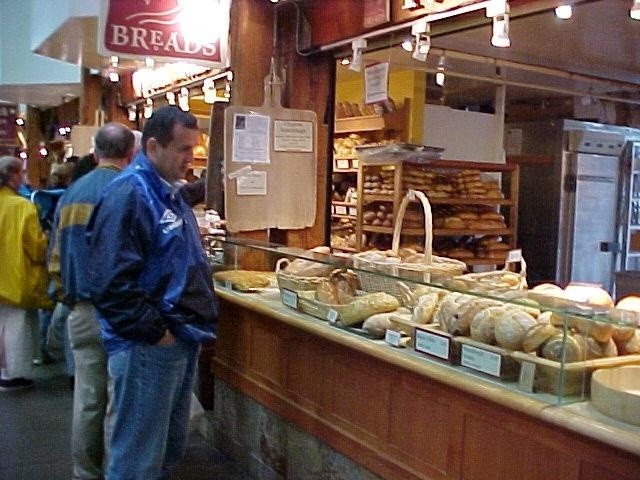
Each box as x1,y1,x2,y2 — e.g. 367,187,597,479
0,375,38,391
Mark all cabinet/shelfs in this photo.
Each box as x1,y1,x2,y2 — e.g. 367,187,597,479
356,157,521,274
204,236,640,480
327,98,411,253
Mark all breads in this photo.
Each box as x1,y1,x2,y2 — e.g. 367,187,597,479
212,269,271,293
284,245,640,369
327,134,513,261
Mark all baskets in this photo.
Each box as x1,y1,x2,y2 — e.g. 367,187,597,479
388,313,640,397
296,289,351,321
353,189,467,306
431,254,529,301
275,258,331,312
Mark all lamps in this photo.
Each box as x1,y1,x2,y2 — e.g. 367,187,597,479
434,54,447,85
126,72,231,121
322,0,640,72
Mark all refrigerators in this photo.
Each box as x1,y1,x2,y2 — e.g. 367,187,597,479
568,130,625,292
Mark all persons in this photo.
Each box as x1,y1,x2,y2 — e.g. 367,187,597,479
1,155,49,390
88,105,223,479
19,153,208,392
50,120,136,480
